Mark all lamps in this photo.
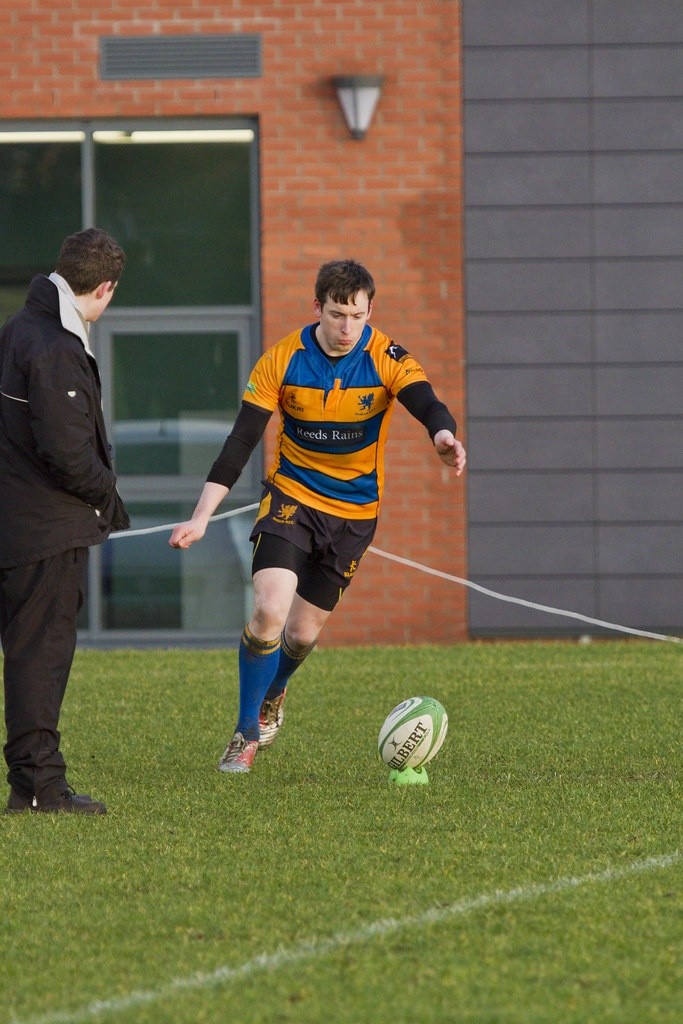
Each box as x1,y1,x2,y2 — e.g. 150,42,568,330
330,75,384,138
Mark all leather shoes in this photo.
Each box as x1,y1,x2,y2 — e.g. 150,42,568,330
4,786,106,815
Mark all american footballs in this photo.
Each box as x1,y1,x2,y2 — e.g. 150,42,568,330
377,696,449,771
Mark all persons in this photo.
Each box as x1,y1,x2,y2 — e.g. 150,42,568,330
168,259,467,775
0,226,130,818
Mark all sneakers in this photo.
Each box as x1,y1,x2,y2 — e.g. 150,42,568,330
258,688,286,748
219,732,258,773
